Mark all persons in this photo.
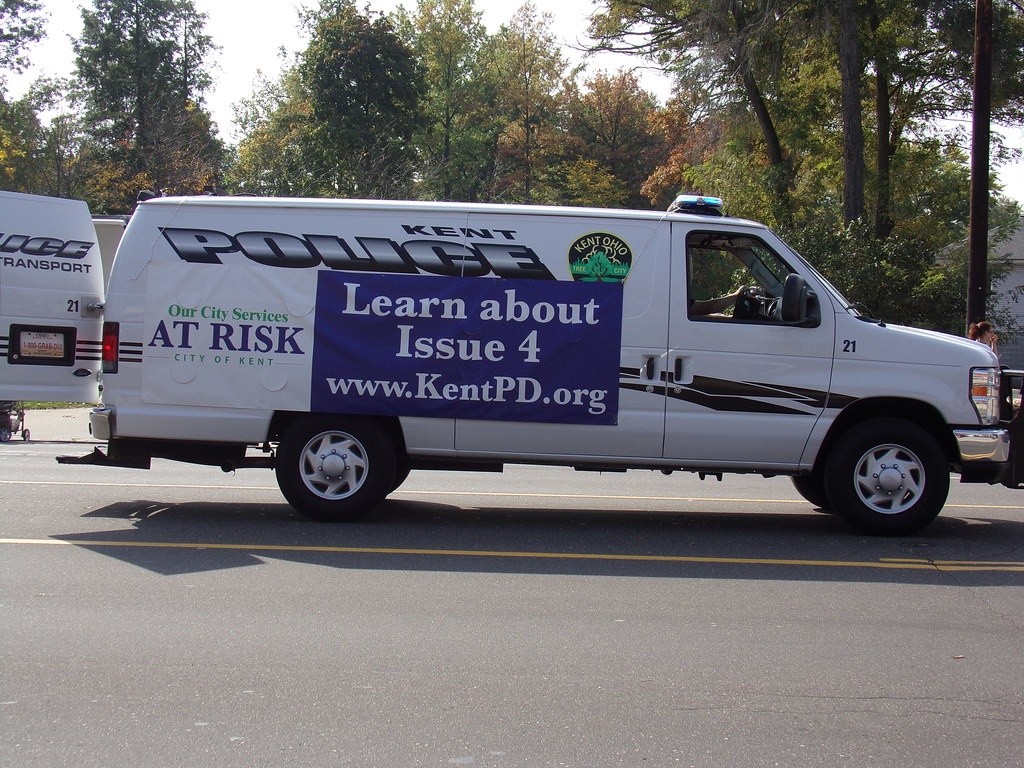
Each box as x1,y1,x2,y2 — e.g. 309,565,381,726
968,321,1000,360
689,285,751,316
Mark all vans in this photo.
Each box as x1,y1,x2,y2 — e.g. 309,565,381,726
0,194,1011,542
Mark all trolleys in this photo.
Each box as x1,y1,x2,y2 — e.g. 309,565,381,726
0,399,31,443
